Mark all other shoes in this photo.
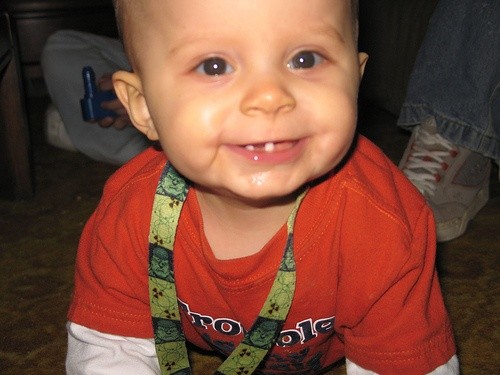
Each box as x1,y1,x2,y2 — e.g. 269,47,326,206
45,105,80,153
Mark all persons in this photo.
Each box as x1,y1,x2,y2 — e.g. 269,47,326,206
40,29,163,179
395,0,500,243
64,0,460,374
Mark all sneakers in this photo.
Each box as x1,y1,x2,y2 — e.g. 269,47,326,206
397,122,491,244
